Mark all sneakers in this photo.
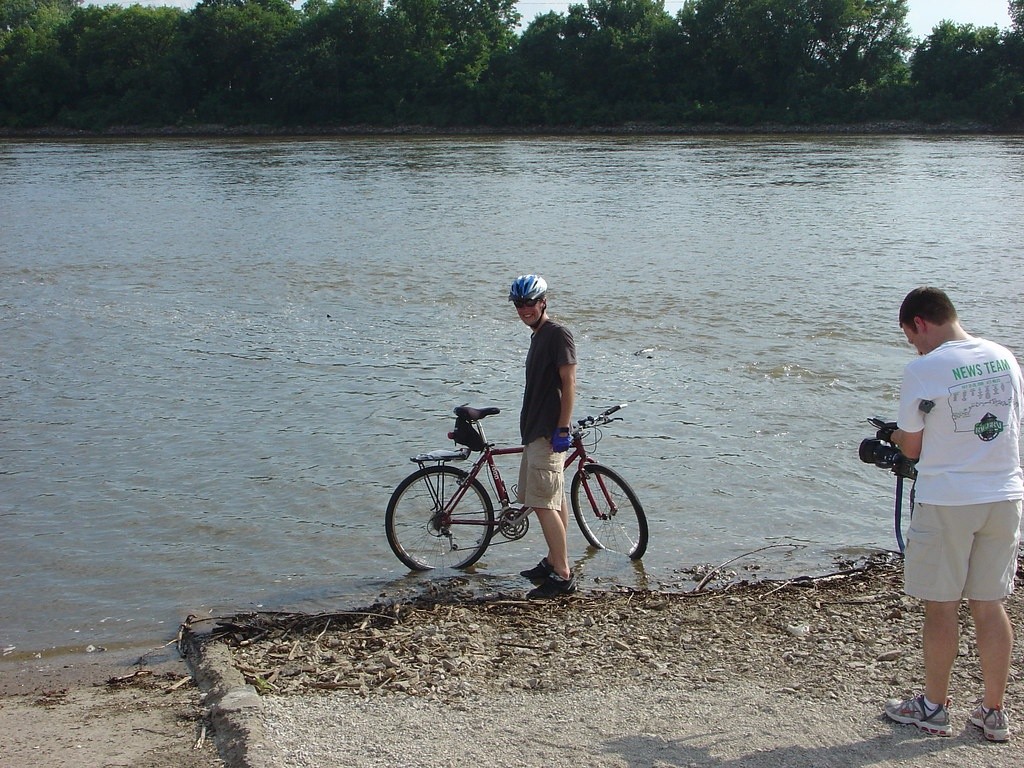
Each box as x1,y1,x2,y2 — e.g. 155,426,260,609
969,701,1010,740
526,568,578,599
520,557,552,578
884,693,952,737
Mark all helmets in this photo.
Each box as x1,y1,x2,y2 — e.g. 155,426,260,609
509,274,547,301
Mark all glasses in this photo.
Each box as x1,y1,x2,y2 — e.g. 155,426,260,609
514,299,540,308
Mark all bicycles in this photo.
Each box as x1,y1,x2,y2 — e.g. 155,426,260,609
384,403,649,572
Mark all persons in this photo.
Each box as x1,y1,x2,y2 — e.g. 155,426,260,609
883,284,1023,743
507,273,578,601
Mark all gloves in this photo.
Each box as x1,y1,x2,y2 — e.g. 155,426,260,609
551,427,571,453
